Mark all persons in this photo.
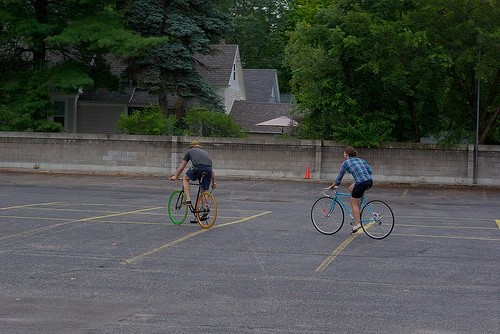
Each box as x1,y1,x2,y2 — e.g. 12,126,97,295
171,140,216,223
327,146,373,233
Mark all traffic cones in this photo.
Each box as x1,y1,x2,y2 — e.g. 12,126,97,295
303,163,313,179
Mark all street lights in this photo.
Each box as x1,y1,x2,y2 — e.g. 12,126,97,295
472,34,486,185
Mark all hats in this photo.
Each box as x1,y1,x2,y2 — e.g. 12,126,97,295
188,140,202,149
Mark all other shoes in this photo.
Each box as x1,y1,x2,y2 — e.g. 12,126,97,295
350,223,362,233
183,200,191,206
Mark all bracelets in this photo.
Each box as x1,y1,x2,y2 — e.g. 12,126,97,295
212,183,216,184
330,185,333,189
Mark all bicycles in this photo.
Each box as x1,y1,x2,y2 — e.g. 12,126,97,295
168,172,217,230
310,186,394,240
